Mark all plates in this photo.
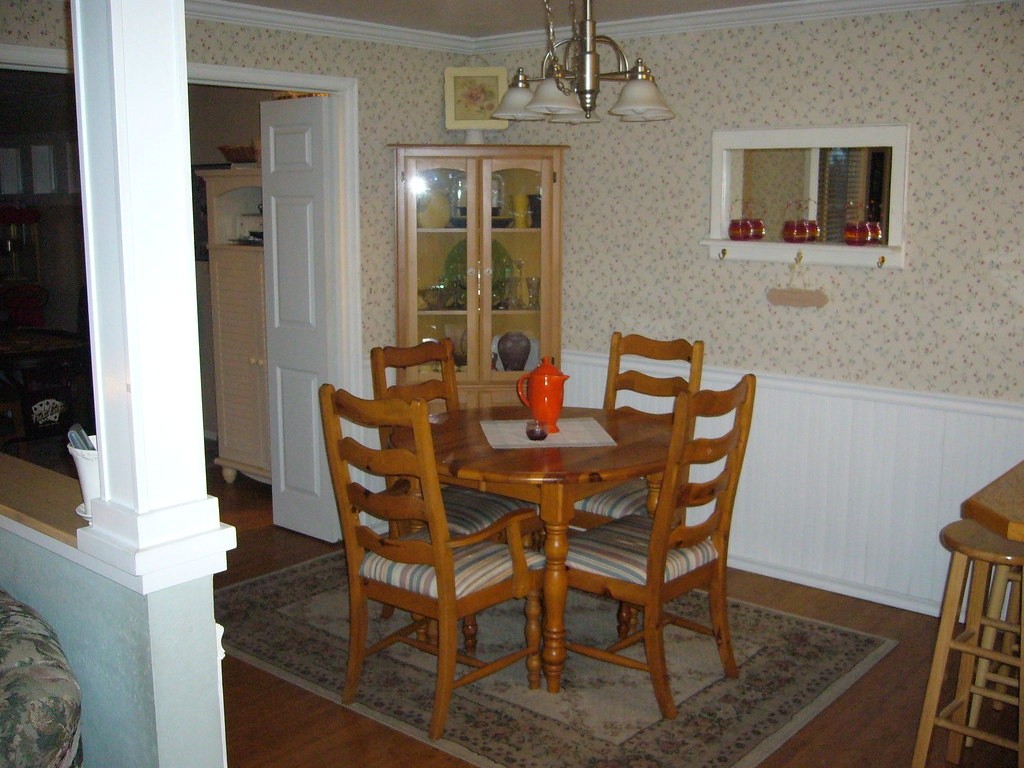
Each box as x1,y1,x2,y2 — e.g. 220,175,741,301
75,503,93,525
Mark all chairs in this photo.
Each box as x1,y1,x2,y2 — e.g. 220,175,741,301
0,400,27,459
568,331,704,633
370,336,544,643
541,374,756,719
319,383,547,740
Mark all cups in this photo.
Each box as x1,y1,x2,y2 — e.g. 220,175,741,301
527,194,542,229
525,416,548,440
510,192,531,229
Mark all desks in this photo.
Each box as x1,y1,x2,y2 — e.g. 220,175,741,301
1,334,91,454
390,406,675,695
960,461,1024,543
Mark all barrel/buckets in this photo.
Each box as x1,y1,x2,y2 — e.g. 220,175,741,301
67,436,102,513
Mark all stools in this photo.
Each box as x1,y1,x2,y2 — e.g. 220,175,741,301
910,520,1024,768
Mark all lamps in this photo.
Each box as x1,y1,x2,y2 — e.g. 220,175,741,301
490,0,674,126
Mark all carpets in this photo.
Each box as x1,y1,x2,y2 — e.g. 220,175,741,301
213,549,899,768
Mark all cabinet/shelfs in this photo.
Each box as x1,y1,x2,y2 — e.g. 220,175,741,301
195,168,273,483
387,144,572,416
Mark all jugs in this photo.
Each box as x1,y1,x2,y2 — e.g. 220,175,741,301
516,355,570,433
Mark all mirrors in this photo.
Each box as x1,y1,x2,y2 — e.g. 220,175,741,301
699,123,910,269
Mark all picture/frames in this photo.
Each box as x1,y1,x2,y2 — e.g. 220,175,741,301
444,66,508,131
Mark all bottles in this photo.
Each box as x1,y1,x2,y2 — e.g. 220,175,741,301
866,222,883,243
783,221,810,243
843,221,871,246
750,218,766,240
798,218,820,240
729,218,753,241
497,329,531,372
